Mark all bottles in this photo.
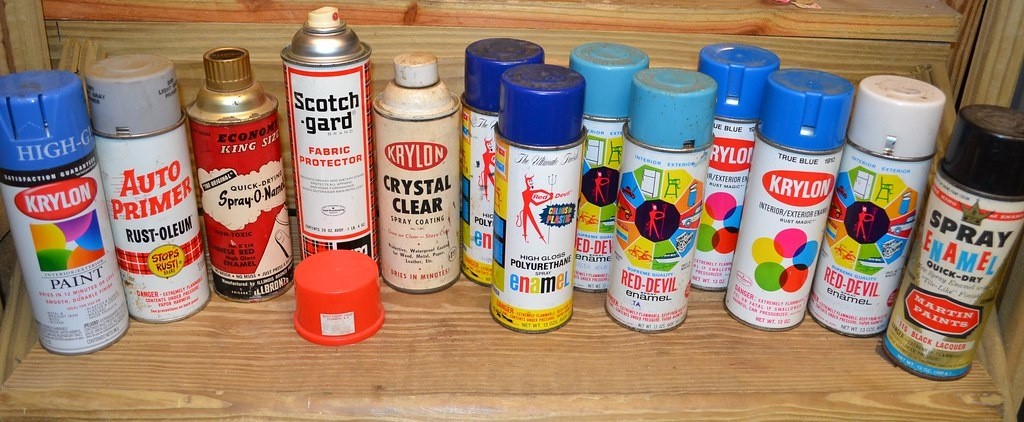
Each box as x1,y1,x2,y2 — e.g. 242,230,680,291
84,55,209,323
492,62,586,331
887,106,1024,381
606,69,718,332
808,74,945,338
0,71,130,353
726,69,854,331
459,41,544,285
570,41,651,289
691,43,781,301
282,5,377,277
371,52,462,292
184,48,295,302
